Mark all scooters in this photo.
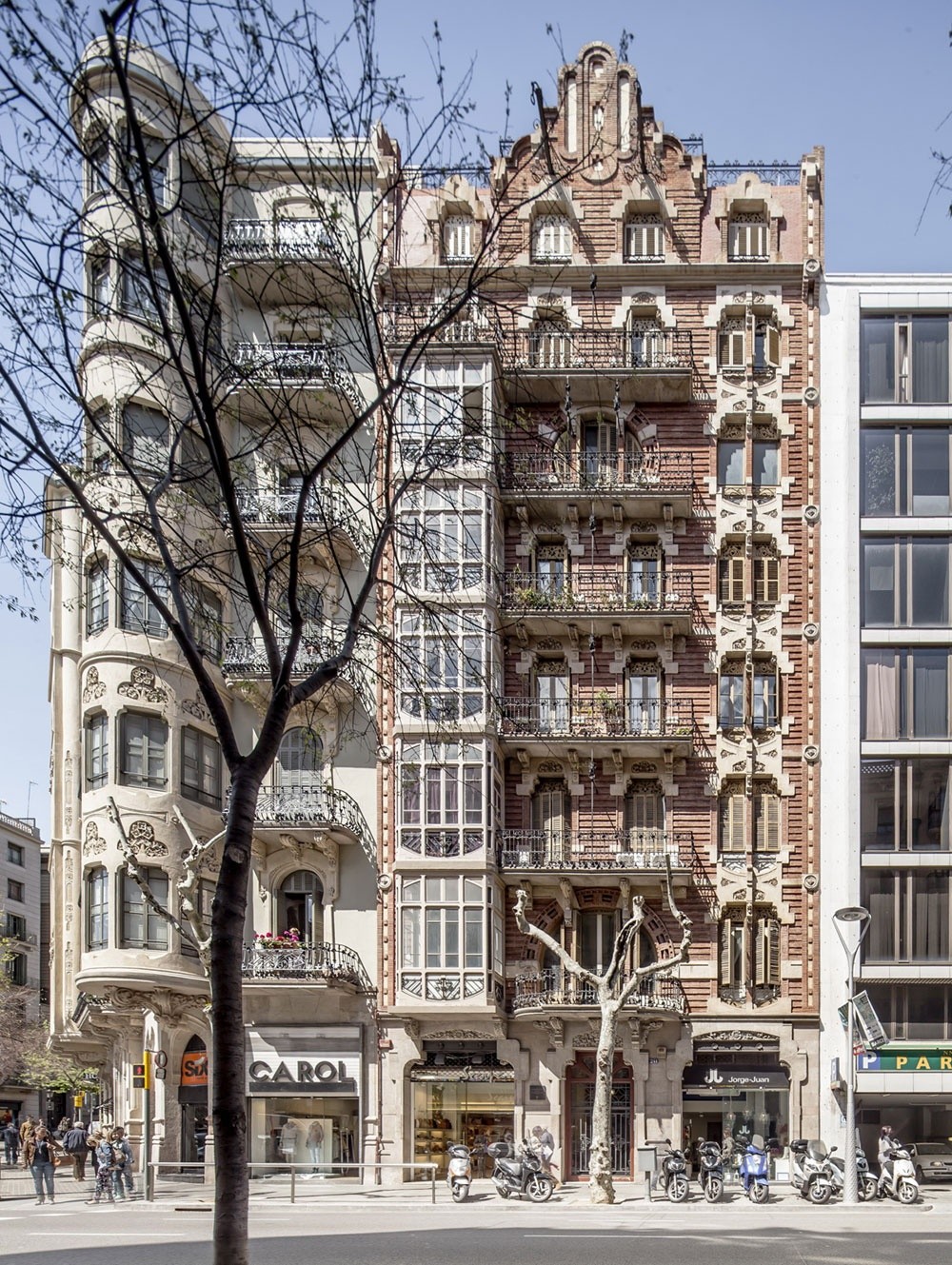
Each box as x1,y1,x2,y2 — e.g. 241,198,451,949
445,1141,480,1203
824,1145,879,1202
696,1137,731,1203
731,1134,772,1204
789,1139,838,1204
877,1138,920,1204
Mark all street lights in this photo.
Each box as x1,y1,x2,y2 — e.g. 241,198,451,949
832,906,872,1204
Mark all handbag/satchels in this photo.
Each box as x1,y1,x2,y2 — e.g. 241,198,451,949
49,1139,61,1167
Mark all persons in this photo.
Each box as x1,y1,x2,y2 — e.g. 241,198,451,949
84,1130,119,1205
872,1125,897,1198
473,1130,488,1178
61,1122,90,1183
529,1125,553,1172
111,1127,137,1193
304,1121,324,1173
0,1107,72,1166
21,1125,64,1205
279,1117,301,1176
104,1128,126,1202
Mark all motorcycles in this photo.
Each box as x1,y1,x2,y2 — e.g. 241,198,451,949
659,1138,692,1203
487,1138,557,1203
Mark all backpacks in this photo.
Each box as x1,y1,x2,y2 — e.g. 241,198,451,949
103,1142,125,1170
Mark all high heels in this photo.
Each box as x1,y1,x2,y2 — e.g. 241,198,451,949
48,1200,56,1205
34,1200,45,1206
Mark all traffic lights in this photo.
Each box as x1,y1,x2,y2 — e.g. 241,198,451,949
134,1065,145,1076
133,1077,145,1089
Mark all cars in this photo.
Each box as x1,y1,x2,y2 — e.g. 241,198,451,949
903,1143,952,1184
